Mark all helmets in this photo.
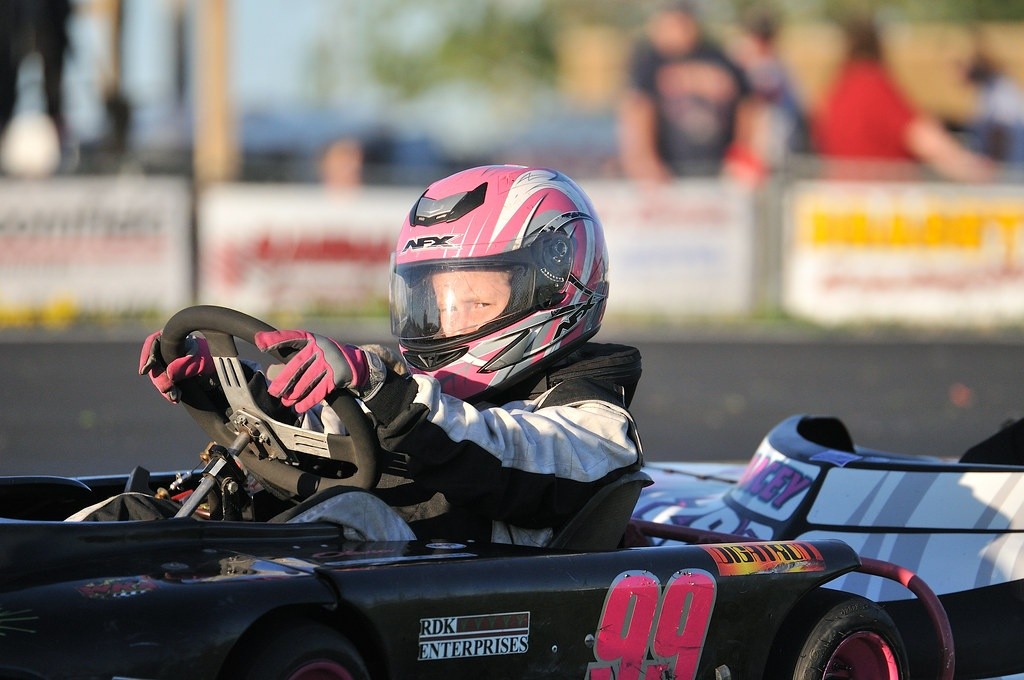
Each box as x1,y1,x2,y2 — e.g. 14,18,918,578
382,162,610,402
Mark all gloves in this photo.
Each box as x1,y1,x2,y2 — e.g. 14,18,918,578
256,328,384,415
140,333,255,413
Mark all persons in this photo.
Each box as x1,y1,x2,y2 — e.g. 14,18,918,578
77,0,1024,191
61,165,645,548
